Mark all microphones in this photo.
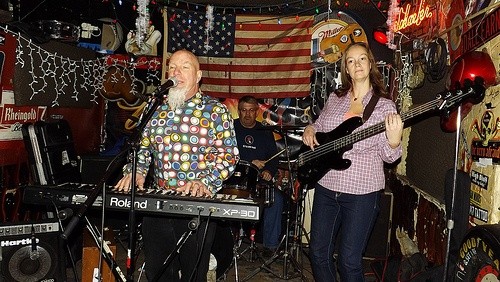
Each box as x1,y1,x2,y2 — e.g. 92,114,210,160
153,77,178,96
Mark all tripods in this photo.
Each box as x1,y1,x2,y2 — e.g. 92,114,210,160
238,224,267,263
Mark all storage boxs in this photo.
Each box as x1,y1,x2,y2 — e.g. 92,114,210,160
81,229,117,282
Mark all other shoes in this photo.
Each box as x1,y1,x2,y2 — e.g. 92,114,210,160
262,248,290,264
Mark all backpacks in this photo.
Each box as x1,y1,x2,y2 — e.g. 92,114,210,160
397,251,445,282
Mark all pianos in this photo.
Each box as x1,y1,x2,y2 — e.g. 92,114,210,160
30,184,263,282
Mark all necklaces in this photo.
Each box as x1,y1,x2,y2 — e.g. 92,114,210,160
354,97,357,101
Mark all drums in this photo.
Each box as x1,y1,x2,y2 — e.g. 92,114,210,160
253,182,279,204
219,158,261,200
173,214,236,282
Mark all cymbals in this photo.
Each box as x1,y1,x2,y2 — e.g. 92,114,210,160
258,124,306,133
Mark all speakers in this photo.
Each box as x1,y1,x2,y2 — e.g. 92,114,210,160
0,232,68,282
333,190,395,261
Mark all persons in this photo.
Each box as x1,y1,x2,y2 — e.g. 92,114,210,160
112,50,239,282
301,43,404,282
231,95,286,265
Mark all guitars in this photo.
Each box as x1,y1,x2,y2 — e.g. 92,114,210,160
296,77,484,188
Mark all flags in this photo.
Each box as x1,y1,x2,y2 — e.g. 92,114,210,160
162,7,314,99
